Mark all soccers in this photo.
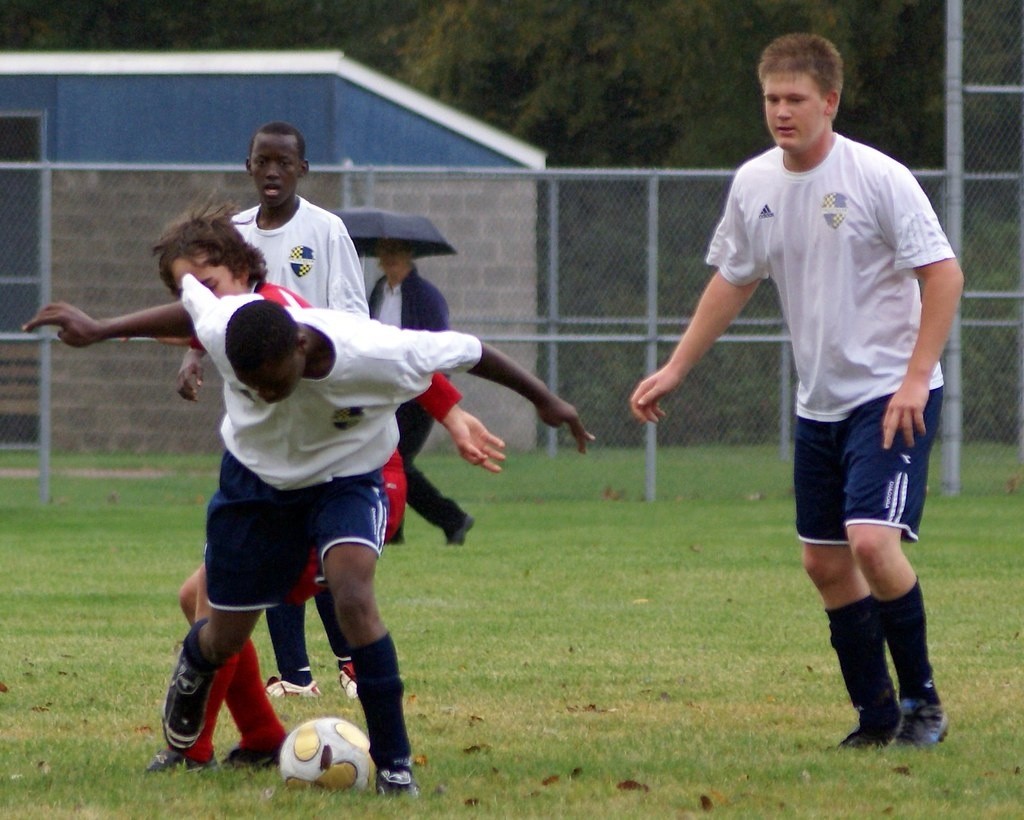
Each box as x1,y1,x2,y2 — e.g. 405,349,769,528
280,716,377,799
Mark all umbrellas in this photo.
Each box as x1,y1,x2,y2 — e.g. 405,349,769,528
331,206,457,275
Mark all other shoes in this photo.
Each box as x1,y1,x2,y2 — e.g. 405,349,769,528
146,749,217,776
447,514,475,545
221,748,280,773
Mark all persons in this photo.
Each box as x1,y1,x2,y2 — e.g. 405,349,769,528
21,121,594,797
628,38,964,750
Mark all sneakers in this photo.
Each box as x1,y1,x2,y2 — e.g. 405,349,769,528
840,706,901,752
162,648,216,752
266,676,322,701
376,768,422,798
339,662,358,699
896,698,948,751
